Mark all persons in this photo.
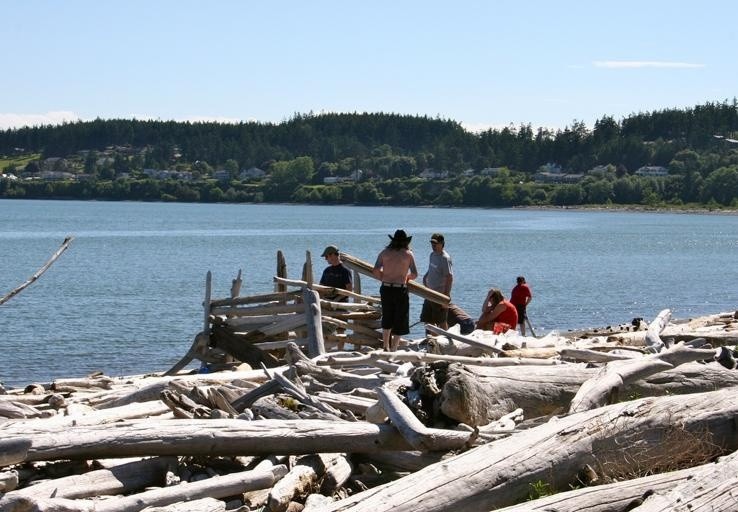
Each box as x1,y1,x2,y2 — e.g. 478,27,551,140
474,288,519,336
374,229,418,351
419,232,453,337
319,245,354,351
446,301,475,337
509,276,532,336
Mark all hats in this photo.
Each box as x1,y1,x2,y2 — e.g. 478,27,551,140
388,230,412,241
321,246,339,257
429,233,444,243
